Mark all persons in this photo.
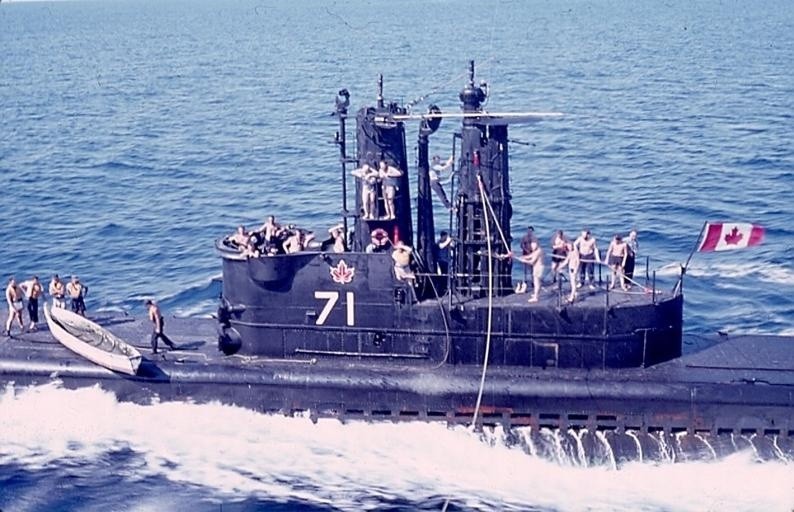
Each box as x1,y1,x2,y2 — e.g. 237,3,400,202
477,237,513,300
144,299,176,352
19,275,44,334
3,276,24,337
514,224,643,302
429,152,458,210
63,273,88,315
226,216,347,255
390,239,418,305
436,227,456,292
349,161,377,220
48,272,66,309
375,159,404,221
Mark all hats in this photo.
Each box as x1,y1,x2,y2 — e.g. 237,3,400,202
70,275,80,283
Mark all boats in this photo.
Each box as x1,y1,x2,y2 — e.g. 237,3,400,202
43,301,141,375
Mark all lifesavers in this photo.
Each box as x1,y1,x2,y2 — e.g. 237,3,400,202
371,229,388,246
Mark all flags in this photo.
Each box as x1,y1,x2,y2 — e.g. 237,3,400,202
693,222,764,256
